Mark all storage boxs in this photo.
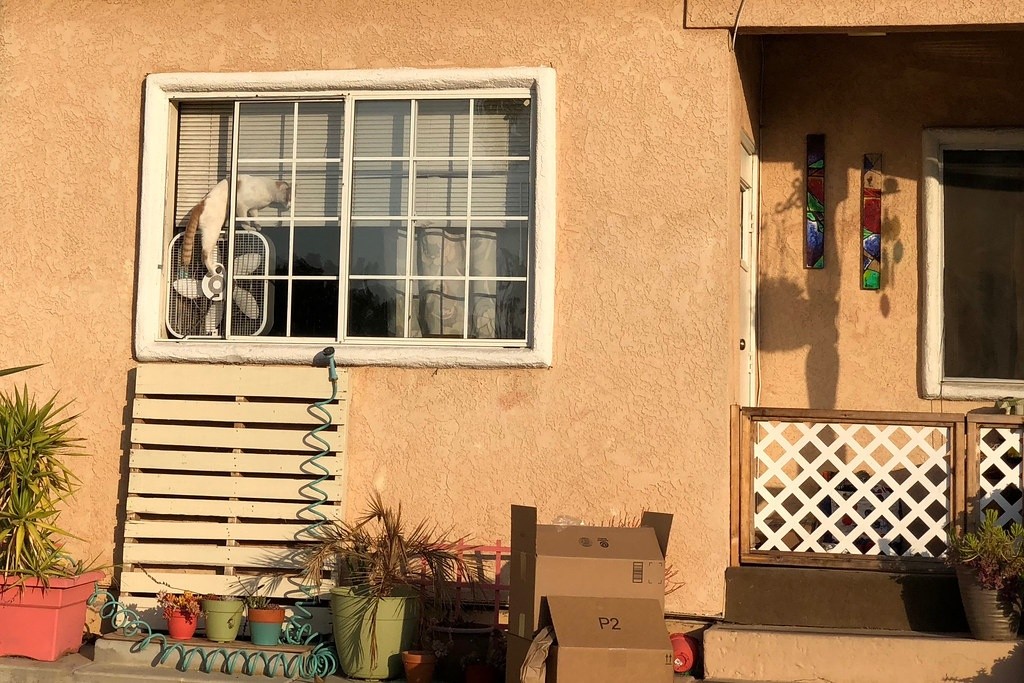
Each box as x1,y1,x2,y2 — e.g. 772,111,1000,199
506,633,533,683
507,505,675,641
533,595,674,683
821,485,903,557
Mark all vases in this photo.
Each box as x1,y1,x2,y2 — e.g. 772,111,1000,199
670,633,699,673
401,650,437,683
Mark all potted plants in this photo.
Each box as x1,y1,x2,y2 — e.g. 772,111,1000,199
247,596,285,645
459,651,494,683
982,397,1023,535
420,609,505,681
950,508,1024,640
202,592,245,643
0,363,133,663
291,487,495,681
156,590,203,640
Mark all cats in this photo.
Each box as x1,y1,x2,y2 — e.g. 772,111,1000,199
180,173,297,277
